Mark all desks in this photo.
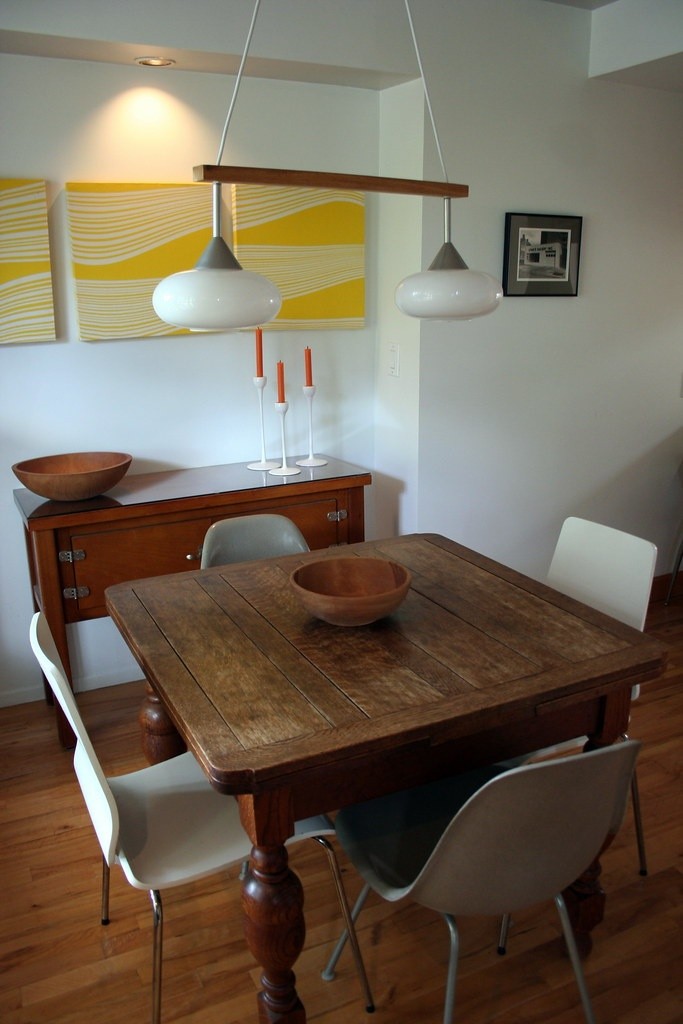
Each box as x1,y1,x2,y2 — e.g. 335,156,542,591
100,532,670,1024
12,453,372,749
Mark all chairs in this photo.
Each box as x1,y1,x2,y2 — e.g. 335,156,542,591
317,737,643,1024
200,512,312,572
495,502,658,879
28,611,376,1024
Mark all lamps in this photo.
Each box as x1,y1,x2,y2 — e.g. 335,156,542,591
151,166,504,333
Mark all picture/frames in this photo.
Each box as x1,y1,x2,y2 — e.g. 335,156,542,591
501,212,583,298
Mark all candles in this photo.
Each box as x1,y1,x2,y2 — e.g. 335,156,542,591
255,327,264,377
277,360,285,403
304,346,312,387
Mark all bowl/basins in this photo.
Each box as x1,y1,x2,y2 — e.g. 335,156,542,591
11,452,132,500
290,558,411,625
